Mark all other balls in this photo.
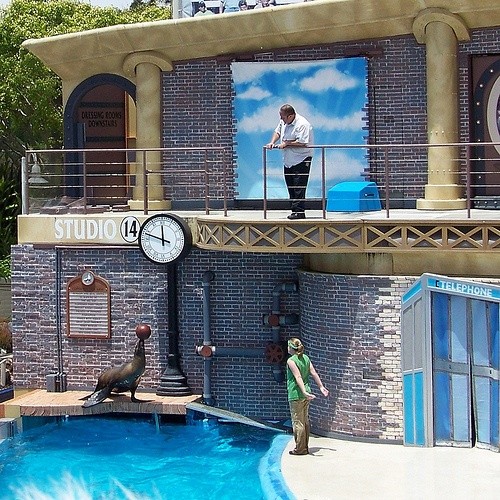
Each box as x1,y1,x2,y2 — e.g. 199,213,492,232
135,325,152,340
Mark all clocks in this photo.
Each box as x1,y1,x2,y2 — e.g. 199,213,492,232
136,213,193,265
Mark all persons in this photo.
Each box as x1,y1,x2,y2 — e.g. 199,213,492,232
267,105,314,219
286,338,329,455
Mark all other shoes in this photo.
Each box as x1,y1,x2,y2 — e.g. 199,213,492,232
289,451,294,455
287,212,306,220
